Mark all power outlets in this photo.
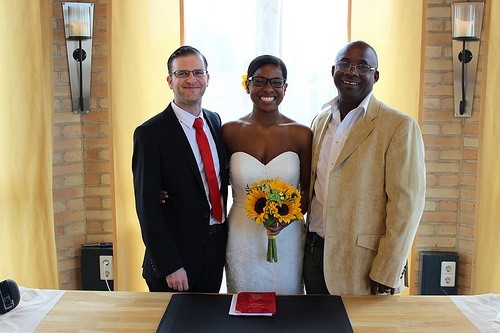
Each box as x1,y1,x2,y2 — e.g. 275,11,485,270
440,261,456,287
100,255,113,280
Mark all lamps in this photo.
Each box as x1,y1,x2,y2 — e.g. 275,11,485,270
62,2,95,114
450,2,484,118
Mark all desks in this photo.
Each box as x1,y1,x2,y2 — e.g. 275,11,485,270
0,289,500,333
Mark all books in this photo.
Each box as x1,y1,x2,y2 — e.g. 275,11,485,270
229,292,277,317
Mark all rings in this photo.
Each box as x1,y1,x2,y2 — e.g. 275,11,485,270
385,289,390,292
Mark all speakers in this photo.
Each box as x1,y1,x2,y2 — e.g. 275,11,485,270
80,245,114,291
417,250,460,296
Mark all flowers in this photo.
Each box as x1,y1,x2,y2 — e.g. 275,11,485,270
242,75,247,86
244,179,304,263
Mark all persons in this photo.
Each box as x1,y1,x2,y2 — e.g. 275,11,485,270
159,56,313,295
303,41,427,296
132,46,231,293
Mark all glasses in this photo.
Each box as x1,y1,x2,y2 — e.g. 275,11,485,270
170,69,207,78
334,62,376,75
248,76,286,88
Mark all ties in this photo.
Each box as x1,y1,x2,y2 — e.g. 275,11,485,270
193,117,222,223
